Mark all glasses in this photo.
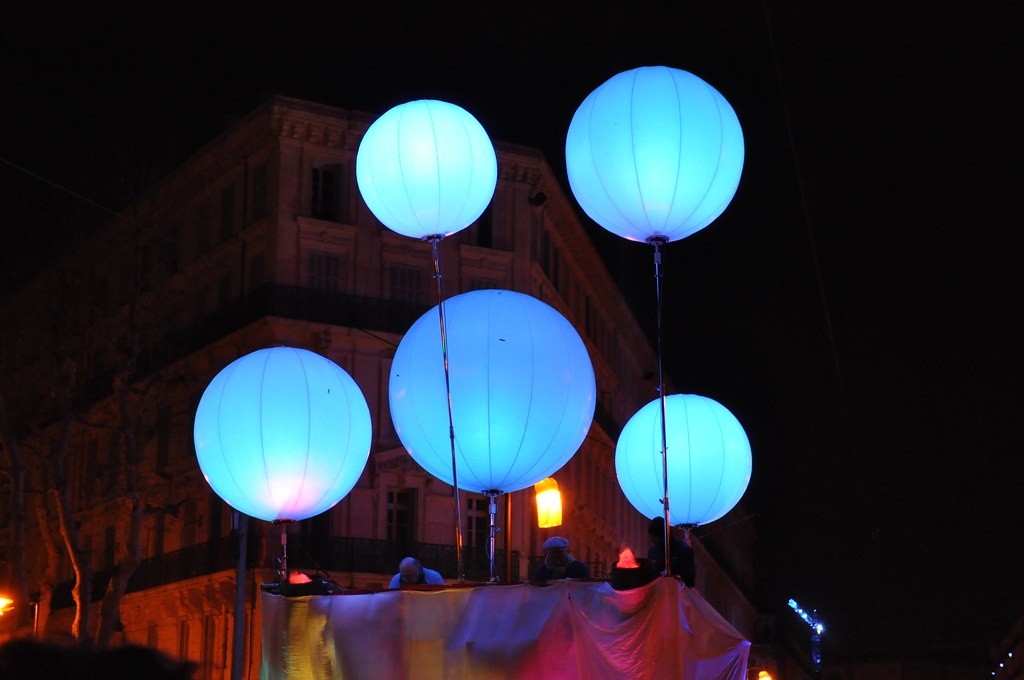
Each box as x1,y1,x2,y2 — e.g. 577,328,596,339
401,570,419,578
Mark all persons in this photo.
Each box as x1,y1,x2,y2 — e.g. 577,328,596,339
388,557,446,589
646,517,698,589
529,537,590,587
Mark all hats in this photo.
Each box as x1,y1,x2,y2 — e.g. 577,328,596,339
544,536,568,550
649,517,664,532
